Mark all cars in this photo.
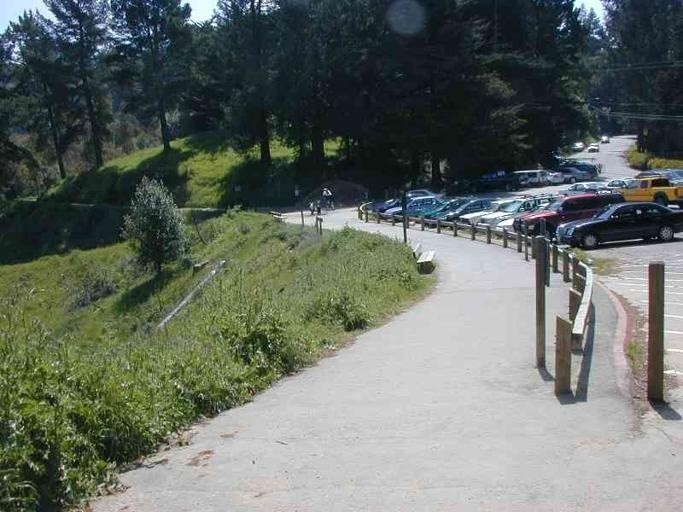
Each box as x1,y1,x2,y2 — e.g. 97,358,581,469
367,189,563,239
479,157,601,192
566,178,635,192
601,135,609,143
589,143,599,152
571,142,585,151
556,203,682,250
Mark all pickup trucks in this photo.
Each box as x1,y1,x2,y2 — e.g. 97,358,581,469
614,176,683,210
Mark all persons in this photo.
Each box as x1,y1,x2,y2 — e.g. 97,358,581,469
306,185,335,215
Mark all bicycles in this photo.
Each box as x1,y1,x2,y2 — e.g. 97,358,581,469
325,196,331,211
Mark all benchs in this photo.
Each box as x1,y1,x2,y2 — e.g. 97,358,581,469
413,244,435,273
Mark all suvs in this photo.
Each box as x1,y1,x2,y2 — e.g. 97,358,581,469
512,195,625,245
